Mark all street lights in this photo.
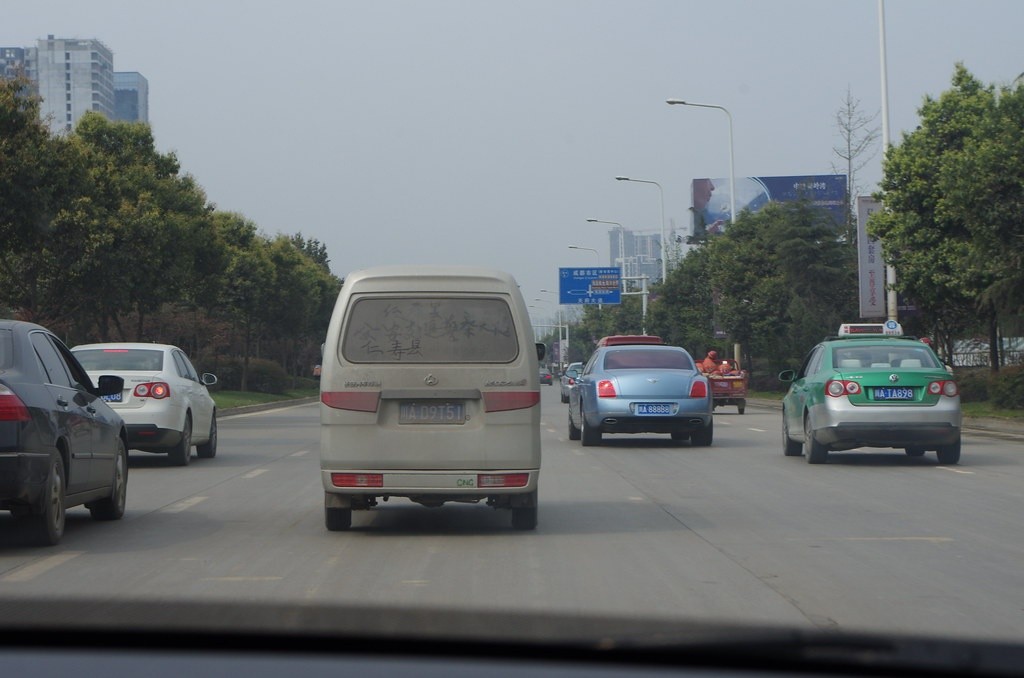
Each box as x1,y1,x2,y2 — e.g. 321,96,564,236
615,175,666,283
527,288,563,377
586,218,627,293
667,98,742,371
567,245,602,308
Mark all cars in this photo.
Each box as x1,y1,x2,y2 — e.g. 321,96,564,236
561,362,586,404
539,367,553,386
568,344,714,446
0,318,131,547
777,322,962,466
69,341,218,466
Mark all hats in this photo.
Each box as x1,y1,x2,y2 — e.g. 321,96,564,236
708,351,717,358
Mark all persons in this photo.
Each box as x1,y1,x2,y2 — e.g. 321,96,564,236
693,178,725,235
697,351,739,377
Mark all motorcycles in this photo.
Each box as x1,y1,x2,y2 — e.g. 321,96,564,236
692,358,749,415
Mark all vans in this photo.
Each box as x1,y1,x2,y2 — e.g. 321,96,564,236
596,335,662,344
317,264,549,530
